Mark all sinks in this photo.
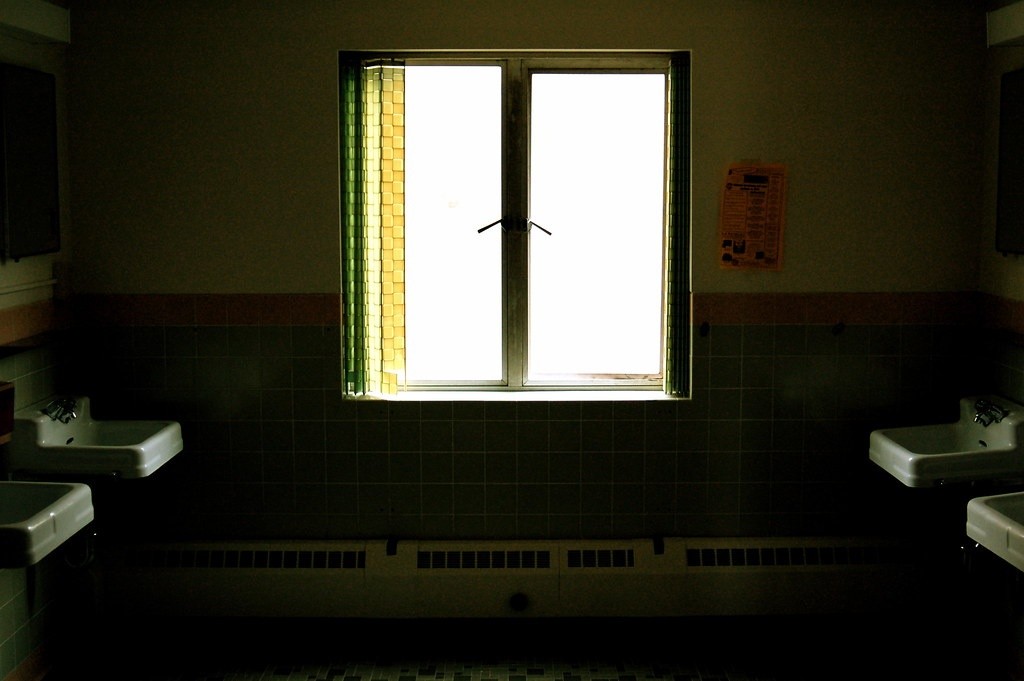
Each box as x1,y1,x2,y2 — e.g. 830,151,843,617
10,395,184,479
0,481,95,570
869,395,1024,487
965,491,1024,573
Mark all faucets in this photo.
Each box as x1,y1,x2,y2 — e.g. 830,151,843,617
46,397,79,424
972,394,1009,427
